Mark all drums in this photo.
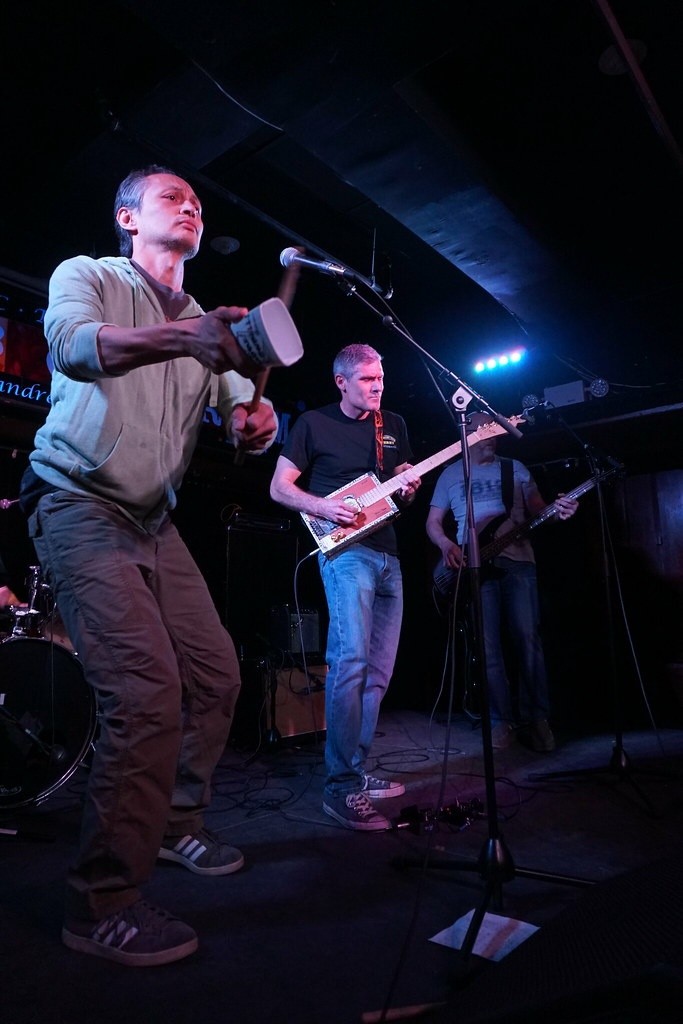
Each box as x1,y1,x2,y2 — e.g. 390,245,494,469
0,628,102,811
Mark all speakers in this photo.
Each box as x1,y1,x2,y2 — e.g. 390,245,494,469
232,656,329,746
261,603,323,656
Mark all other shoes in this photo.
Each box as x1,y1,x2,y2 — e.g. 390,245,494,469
491,720,519,748
528,718,553,751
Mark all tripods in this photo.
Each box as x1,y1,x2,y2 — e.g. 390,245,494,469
333,274,610,993
528,404,683,823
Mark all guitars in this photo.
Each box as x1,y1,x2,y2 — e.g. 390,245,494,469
432,458,625,597
300,399,550,557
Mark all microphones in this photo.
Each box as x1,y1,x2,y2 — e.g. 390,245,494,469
279,247,356,282
523,400,552,416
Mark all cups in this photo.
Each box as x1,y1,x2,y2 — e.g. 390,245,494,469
227,298,305,371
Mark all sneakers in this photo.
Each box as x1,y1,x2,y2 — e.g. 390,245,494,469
322,786,391,833
62,897,198,967
158,827,244,876
361,774,405,798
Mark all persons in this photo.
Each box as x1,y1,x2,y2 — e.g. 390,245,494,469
426,405,578,753
19,165,282,965
267,341,422,829
